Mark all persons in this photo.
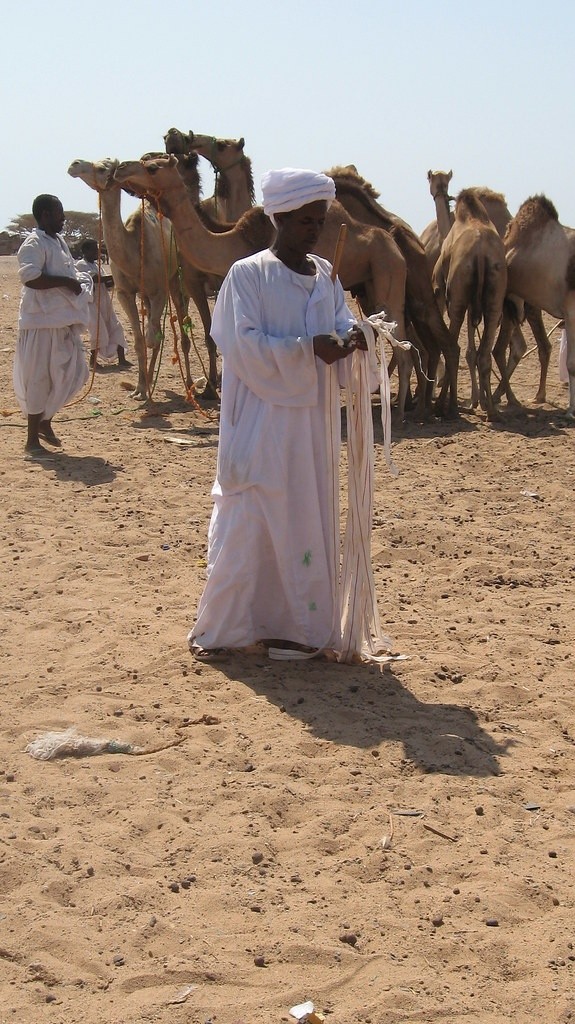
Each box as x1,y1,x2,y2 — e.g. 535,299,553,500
73,239,136,366
185,166,379,661
14,194,95,458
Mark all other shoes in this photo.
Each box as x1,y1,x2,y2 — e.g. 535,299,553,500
118,361,133,366
88,361,94,371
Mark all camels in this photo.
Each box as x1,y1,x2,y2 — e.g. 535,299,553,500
67,127,575,427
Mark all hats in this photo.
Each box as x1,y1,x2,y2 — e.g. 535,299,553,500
261,168,336,230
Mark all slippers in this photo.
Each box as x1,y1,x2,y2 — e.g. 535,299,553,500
190,646,230,661
264,638,319,653
23,447,52,458
38,432,62,447
